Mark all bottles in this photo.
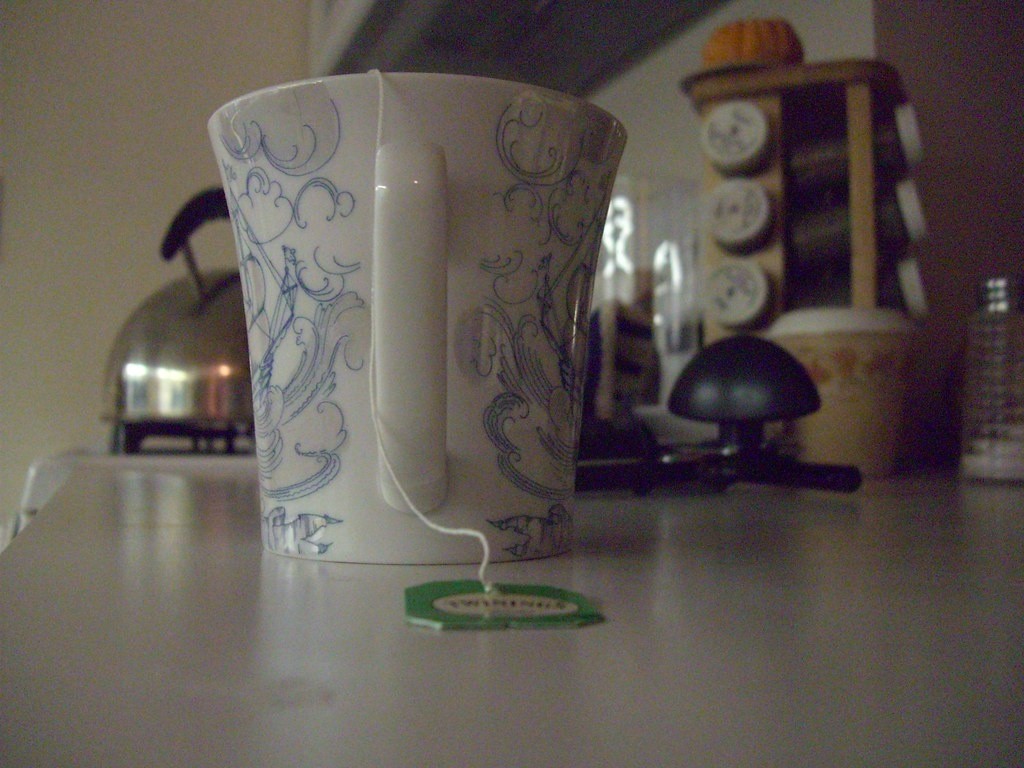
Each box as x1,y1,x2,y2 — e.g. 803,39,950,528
699,99,928,330
957,276,1024,482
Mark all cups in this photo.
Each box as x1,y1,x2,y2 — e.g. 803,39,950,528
771,310,934,478
207,72,629,565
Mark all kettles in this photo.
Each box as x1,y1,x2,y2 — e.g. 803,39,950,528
97,188,255,434
